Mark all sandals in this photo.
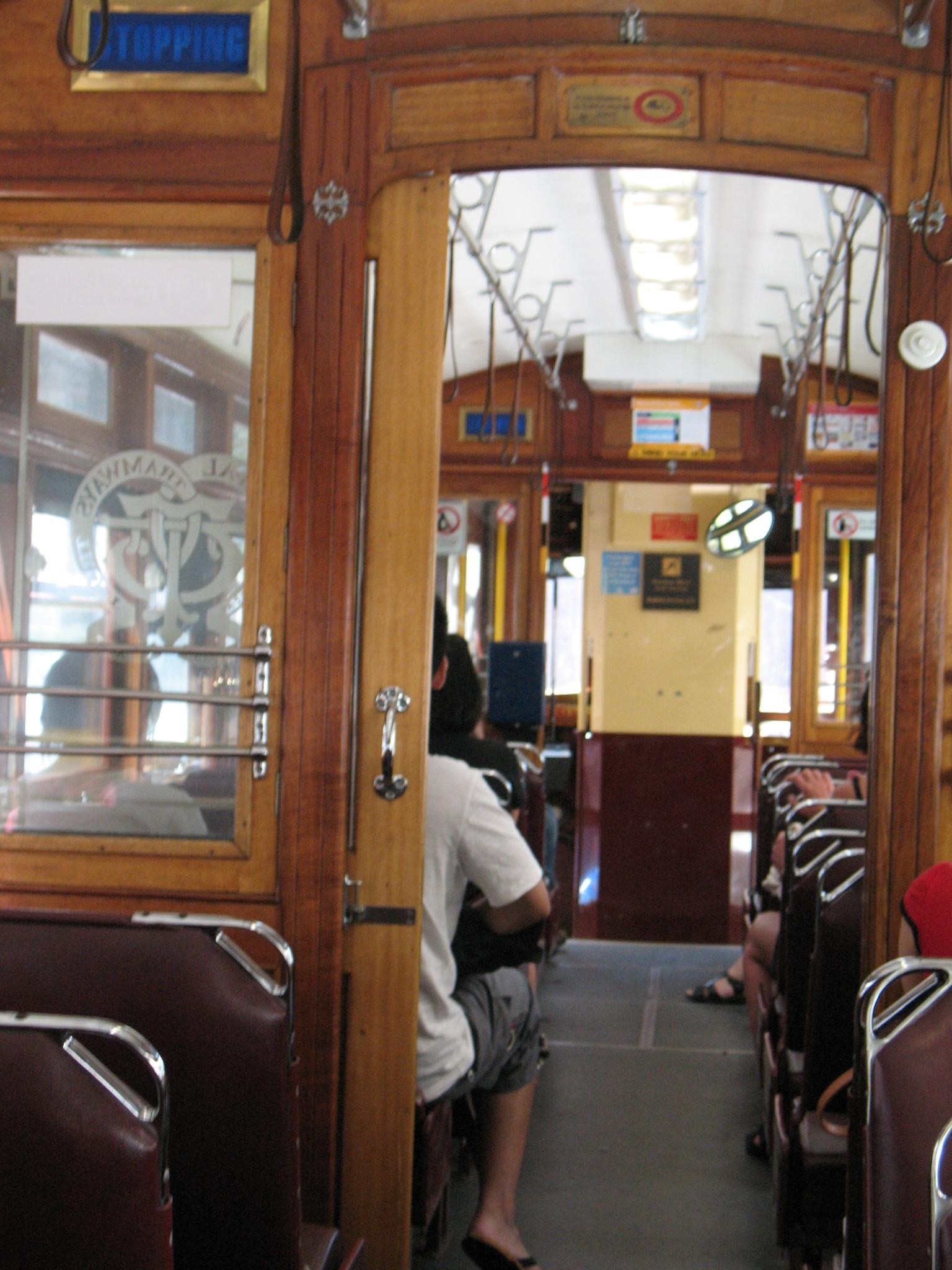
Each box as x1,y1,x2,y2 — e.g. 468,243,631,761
686,971,746,1003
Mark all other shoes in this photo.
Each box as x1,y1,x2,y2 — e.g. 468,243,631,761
748,1131,766,1155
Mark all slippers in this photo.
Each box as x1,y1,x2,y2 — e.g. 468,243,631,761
458,1234,537,1270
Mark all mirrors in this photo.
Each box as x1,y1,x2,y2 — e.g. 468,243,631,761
704,498,775,560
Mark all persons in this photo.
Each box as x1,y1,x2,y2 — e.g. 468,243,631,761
683,686,869,1162
421,591,557,1270
897,860,952,993
0,639,210,839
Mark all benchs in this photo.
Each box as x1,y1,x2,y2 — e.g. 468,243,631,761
0,741,547,1270
751,750,952,1270
